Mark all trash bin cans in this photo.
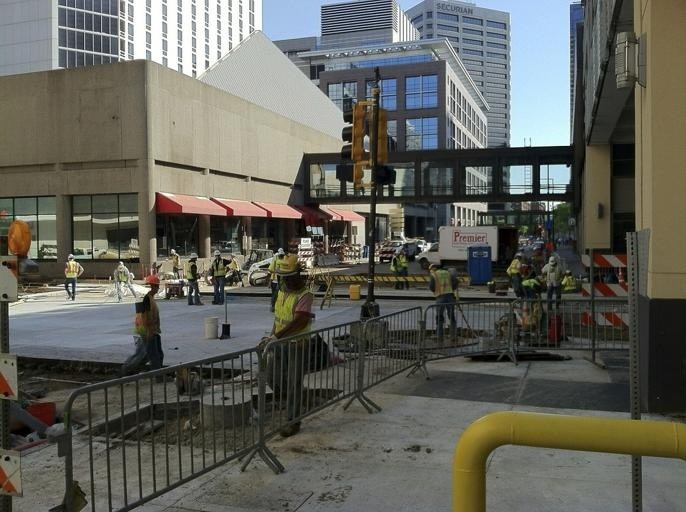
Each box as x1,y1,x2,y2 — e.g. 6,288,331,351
467,245,492,286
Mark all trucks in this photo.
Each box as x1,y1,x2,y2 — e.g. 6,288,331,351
418,225,498,270
378,240,418,263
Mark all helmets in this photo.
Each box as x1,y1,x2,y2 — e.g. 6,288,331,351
67,253,75,260
277,247,285,255
275,253,301,276
214,250,220,258
549,255,557,266
513,252,522,259
171,248,176,254
142,275,162,285
189,252,198,258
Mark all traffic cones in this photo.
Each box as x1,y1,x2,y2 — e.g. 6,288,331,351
519,303,533,332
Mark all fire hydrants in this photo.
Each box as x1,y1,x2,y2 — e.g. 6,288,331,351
550,313,562,347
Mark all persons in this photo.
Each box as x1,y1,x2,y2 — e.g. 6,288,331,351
228,253,244,287
506,253,577,313
256,254,314,438
398,248,409,290
63,254,84,301
170,248,181,279
428,263,459,343
209,250,233,306
265,253,286,313
389,250,402,290
117,274,174,384
185,252,203,306
113,260,137,304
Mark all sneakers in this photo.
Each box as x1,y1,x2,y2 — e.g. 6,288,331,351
118,362,129,378
277,419,301,438
156,374,173,384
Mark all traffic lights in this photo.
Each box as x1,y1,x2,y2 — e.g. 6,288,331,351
335,94,397,194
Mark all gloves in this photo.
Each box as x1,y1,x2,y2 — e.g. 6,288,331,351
256,334,278,351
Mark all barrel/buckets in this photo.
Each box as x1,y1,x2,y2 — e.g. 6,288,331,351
478,337,491,351
203,317,219,338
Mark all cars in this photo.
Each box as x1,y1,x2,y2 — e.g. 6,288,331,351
74,248,108,259
